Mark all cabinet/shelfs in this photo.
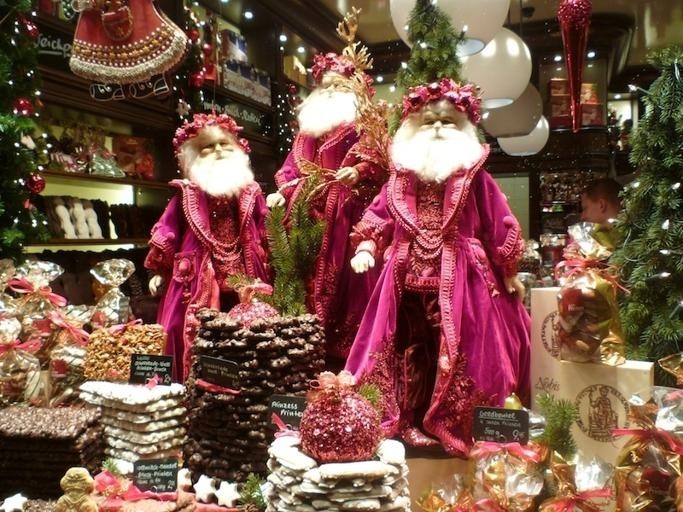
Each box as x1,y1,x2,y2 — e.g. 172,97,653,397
1,0,324,305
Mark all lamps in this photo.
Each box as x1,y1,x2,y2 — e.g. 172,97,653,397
390,0,548,158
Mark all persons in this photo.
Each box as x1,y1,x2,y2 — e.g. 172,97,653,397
579,177,624,226
